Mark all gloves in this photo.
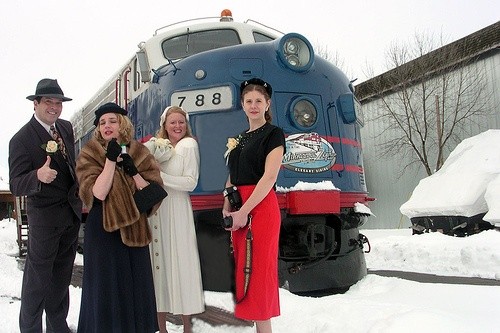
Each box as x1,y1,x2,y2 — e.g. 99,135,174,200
104,138,122,161
117,154,138,177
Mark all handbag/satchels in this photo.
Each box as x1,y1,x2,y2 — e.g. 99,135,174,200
132,182,167,213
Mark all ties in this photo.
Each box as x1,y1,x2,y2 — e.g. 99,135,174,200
49,126,76,183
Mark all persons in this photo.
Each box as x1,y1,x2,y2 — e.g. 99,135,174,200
8,78,83,333
142,105,206,333
74,102,164,333
221,77,287,333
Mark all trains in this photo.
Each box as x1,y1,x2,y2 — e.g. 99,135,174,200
68,7,378,297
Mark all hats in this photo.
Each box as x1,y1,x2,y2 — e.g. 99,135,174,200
94,103,127,125
160,105,190,125
27,79,72,102
240,77,272,97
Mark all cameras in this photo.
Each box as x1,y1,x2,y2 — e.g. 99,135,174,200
219,185,249,229
